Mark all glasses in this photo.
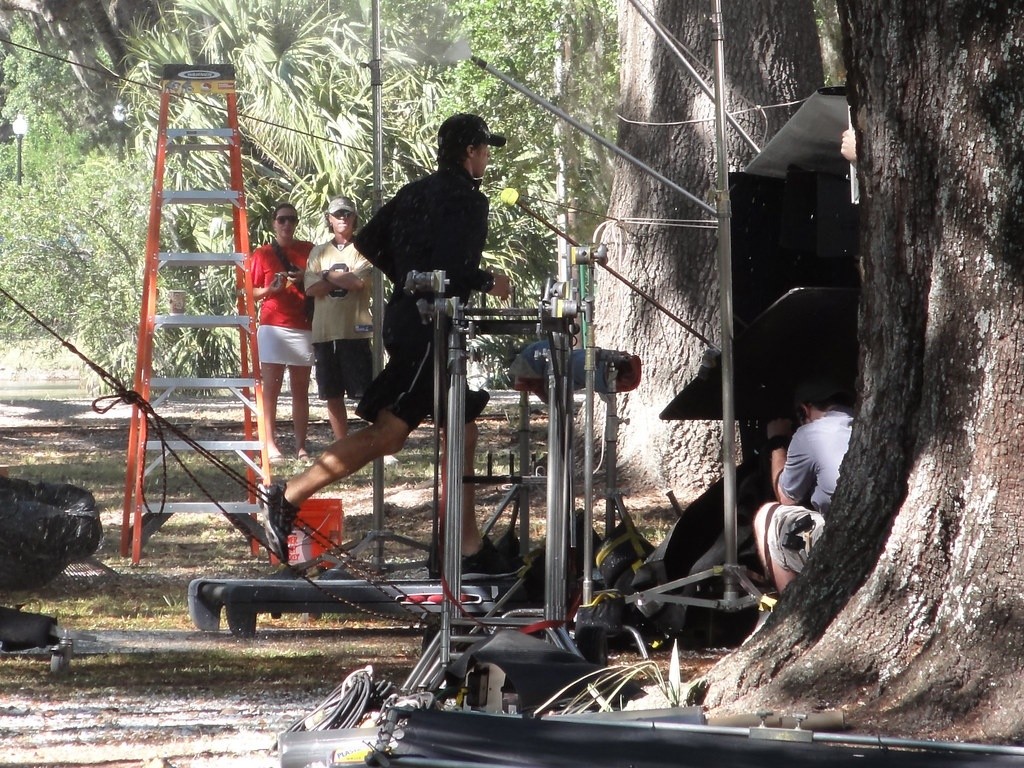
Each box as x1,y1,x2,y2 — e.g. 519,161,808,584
274,216,298,224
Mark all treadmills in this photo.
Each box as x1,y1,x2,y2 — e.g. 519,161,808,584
186,337,641,641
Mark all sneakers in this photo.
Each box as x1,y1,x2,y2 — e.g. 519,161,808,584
256,481,301,563
461,535,526,581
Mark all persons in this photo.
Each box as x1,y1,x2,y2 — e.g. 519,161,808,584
251,204,315,466
257,113,528,582
303,196,400,467
751,374,857,597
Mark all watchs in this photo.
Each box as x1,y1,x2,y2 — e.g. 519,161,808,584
322,270,330,282
484,276,496,293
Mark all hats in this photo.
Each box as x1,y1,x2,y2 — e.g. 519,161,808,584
438,113,507,148
328,197,356,215
794,374,848,411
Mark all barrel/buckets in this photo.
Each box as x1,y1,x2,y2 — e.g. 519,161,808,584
284,499,345,566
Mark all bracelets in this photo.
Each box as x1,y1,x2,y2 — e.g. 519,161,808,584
768,435,790,453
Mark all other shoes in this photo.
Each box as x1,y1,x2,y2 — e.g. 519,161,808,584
268,454,313,466
383,455,399,466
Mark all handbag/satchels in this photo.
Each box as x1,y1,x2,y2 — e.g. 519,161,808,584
303,296,314,323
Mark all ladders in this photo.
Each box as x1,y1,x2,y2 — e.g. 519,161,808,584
121,63,281,567
